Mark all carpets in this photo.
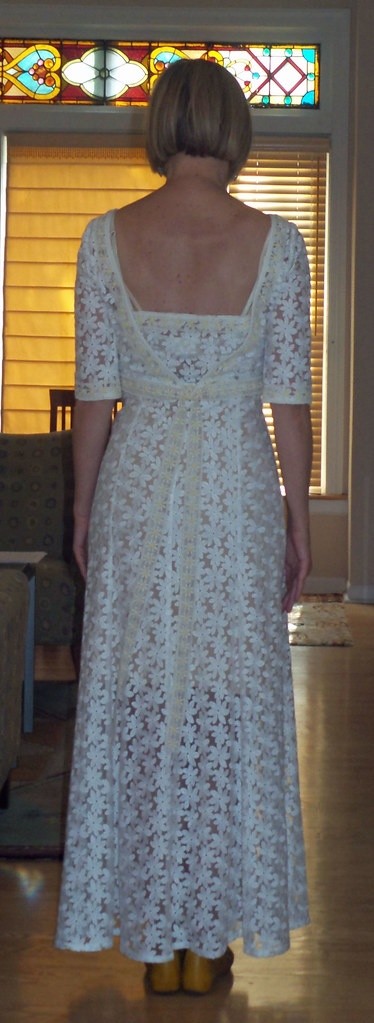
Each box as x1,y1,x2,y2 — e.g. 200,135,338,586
0,679,79,861
288,601,353,647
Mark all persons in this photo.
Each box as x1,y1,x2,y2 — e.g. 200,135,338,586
53,59,312,994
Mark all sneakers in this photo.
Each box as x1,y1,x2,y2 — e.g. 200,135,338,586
147,951,180,992
184,947,234,994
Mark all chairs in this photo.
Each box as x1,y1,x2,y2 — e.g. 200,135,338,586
49,390,124,433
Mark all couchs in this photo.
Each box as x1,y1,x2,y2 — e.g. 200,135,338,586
0,429,87,647
